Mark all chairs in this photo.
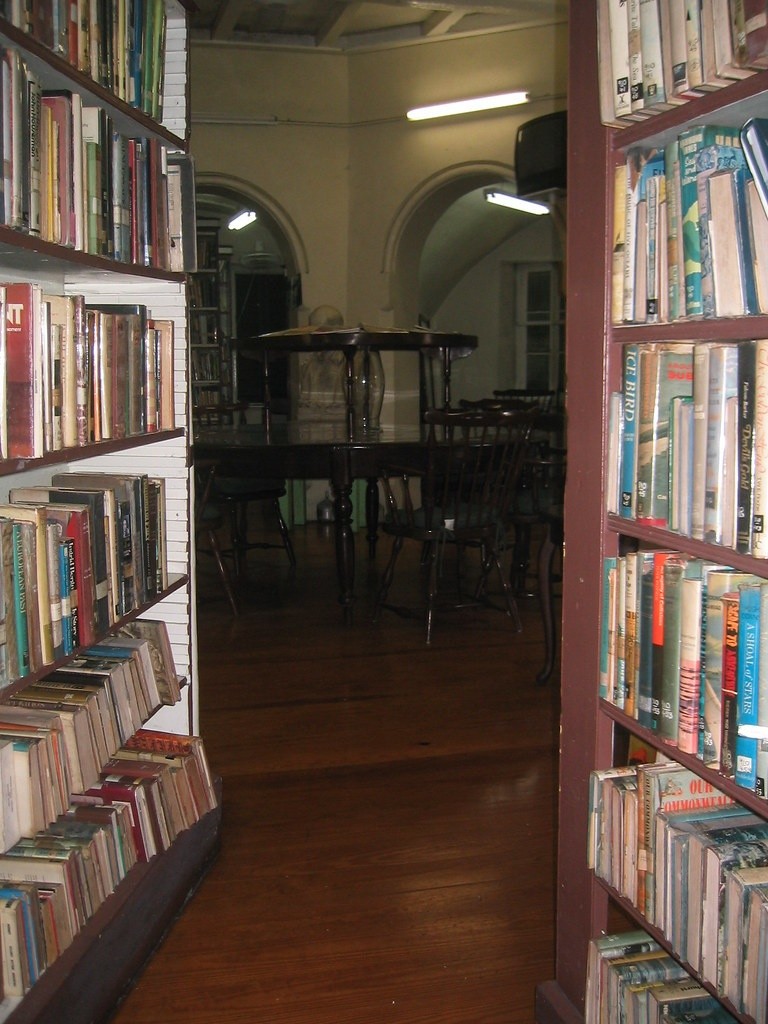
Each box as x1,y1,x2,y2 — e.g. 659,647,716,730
194,449,241,621
192,401,302,584
421,388,568,567
372,401,523,646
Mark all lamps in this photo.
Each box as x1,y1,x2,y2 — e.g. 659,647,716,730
406,90,531,122
240,239,282,270
227,211,258,232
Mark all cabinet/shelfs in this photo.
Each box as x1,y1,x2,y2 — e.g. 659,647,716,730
218,245,233,428
187,216,223,428
0,0,220,1024
535,0,768,1024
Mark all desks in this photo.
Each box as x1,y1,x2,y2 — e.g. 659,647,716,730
194,421,553,632
250,327,480,441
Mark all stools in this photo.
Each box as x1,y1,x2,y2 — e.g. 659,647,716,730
525,459,567,686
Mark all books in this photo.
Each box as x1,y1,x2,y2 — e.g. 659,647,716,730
585,747,768,1024
597,545,768,809
611,109,767,328
596,0,768,131
0,282,175,463
583,930,753,1024
192,349,221,382
1,470,169,696
0,612,182,863
0,0,174,123
191,312,219,344
191,388,227,428
0,47,199,277
1,725,221,1011
196,236,221,272
606,335,767,564
187,275,220,308
